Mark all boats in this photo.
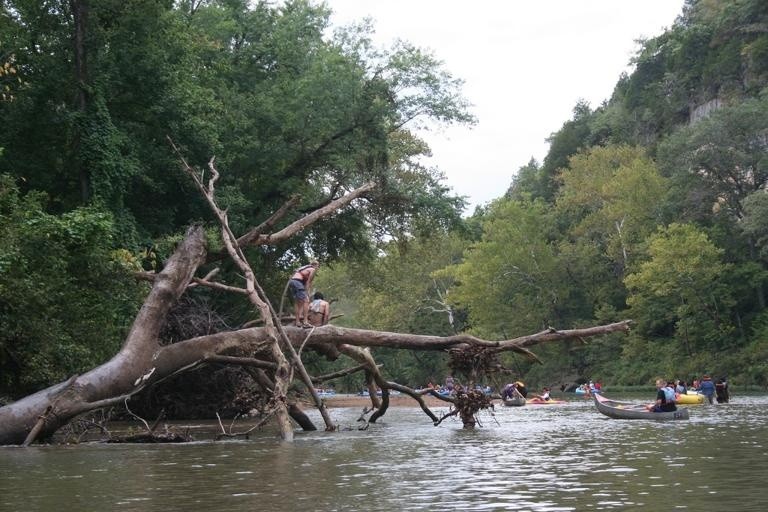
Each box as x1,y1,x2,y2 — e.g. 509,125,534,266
503,387,601,406
594,386,705,421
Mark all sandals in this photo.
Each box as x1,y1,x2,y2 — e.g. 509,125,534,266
295,320,302,328
302,322,314,329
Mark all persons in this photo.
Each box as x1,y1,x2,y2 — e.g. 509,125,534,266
289,260,320,329
371,373,605,400
308,291,351,351
646,373,732,412
